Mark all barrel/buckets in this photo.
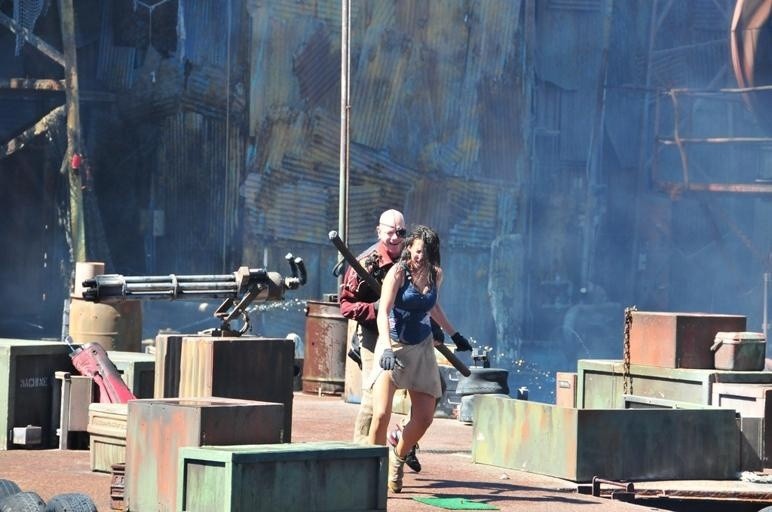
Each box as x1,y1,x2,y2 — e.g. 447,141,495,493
68,295,141,352
302,300,346,396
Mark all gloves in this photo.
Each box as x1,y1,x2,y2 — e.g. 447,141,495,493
450,331,474,352
379,347,406,371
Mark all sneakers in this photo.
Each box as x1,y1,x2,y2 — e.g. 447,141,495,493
388,446,409,494
387,425,422,473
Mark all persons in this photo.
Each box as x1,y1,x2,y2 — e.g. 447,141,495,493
368,223,472,495
338,207,446,474
